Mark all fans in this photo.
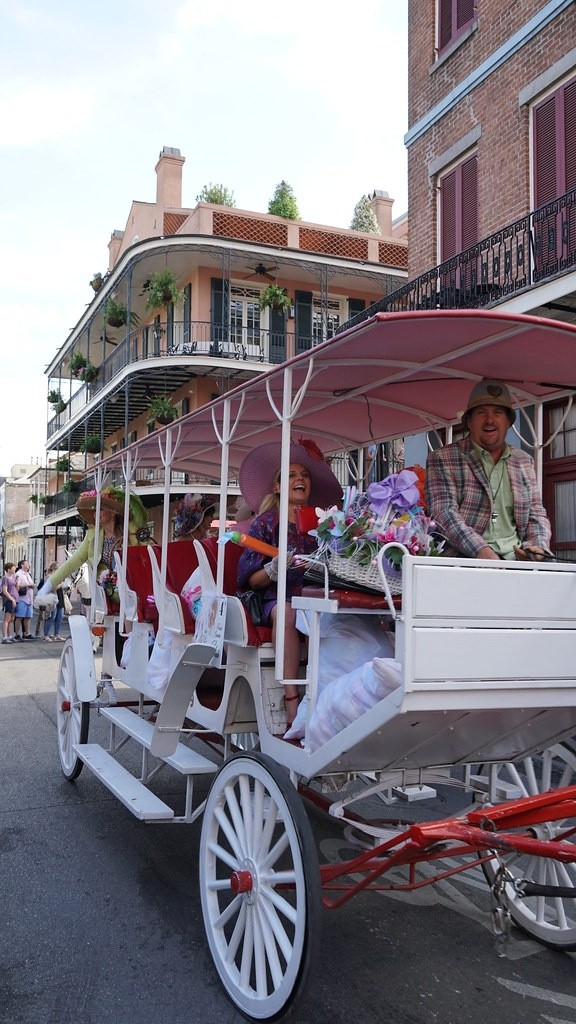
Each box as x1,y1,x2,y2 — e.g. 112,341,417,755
243,263,281,282
94,326,118,346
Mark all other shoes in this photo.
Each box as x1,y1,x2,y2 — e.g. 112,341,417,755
54,636,66,641
43,637,52,642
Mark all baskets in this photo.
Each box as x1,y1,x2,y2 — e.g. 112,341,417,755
329,502,403,596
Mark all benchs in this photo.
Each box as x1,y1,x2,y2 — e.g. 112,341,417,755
88,533,404,754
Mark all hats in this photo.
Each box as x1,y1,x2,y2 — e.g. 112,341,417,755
174,493,220,537
239,436,343,514
77,487,149,528
235,495,254,523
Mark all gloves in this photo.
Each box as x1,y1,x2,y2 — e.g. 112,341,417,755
37,581,53,596
263,551,296,583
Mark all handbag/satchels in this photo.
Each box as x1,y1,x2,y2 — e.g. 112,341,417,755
18,587,27,596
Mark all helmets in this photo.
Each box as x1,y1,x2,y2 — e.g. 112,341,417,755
462,378,516,427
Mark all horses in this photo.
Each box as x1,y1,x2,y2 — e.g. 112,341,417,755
65,549,104,654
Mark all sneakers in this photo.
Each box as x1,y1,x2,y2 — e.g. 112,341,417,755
11,637,17,642
14,635,24,641
24,634,36,640
2,638,12,644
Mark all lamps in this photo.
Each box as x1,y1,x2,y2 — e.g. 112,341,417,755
152,323,166,339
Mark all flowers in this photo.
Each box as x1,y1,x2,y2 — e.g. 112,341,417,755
77,365,96,381
315,505,448,571
171,491,213,539
80,489,97,497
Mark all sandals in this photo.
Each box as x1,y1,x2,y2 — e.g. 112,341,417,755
283,693,300,729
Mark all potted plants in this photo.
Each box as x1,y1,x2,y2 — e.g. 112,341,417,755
138,270,186,313
53,400,67,413
47,391,62,403
259,262,292,312
148,394,177,425
62,479,79,492
102,299,143,328
29,495,37,503
70,354,87,376
56,457,72,472
40,495,52,504
82,433,108,455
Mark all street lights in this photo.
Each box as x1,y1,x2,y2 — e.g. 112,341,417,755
1,529,6,576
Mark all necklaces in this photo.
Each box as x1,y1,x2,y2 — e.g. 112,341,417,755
191,533,196,539
478,456,504,523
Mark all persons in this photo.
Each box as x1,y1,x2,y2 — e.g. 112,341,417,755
0,560,67,642
33,485,156,639
236,436,345,733
171,492,216,541
425,379,557,562
